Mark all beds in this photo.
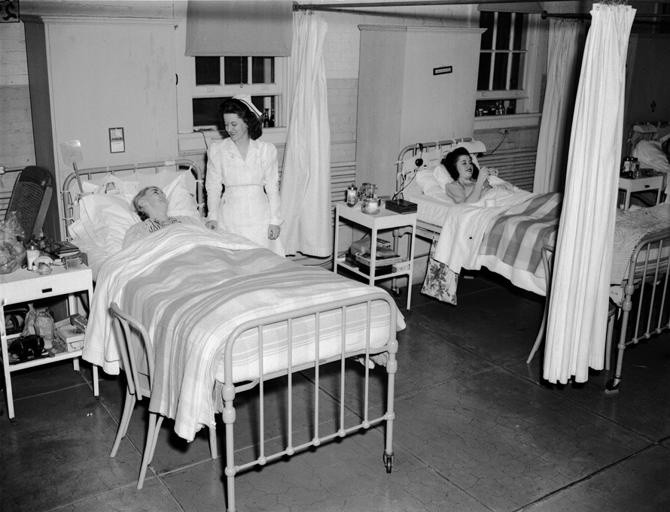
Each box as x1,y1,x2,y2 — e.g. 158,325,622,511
397,139,670,393
61,159,407,511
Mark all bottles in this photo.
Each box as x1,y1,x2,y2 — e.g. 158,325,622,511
34,308,55,350
346,183,357,208
27,245,39,271
475,101,513,116
624,156,640,176
270,109,274,127
264,108,270,127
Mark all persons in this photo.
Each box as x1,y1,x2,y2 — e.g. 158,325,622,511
442,145,501,205
626,124,670,207
205,93,286,259
121,185,204,249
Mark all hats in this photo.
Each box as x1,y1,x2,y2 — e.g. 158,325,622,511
232,93,263,119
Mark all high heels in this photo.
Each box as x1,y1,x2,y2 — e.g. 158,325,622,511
9,334,44,361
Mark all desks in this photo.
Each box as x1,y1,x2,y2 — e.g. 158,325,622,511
618,172,663,210
334,197,417,311
0,249,99,419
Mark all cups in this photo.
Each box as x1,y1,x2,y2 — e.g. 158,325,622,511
38,262,52,276
